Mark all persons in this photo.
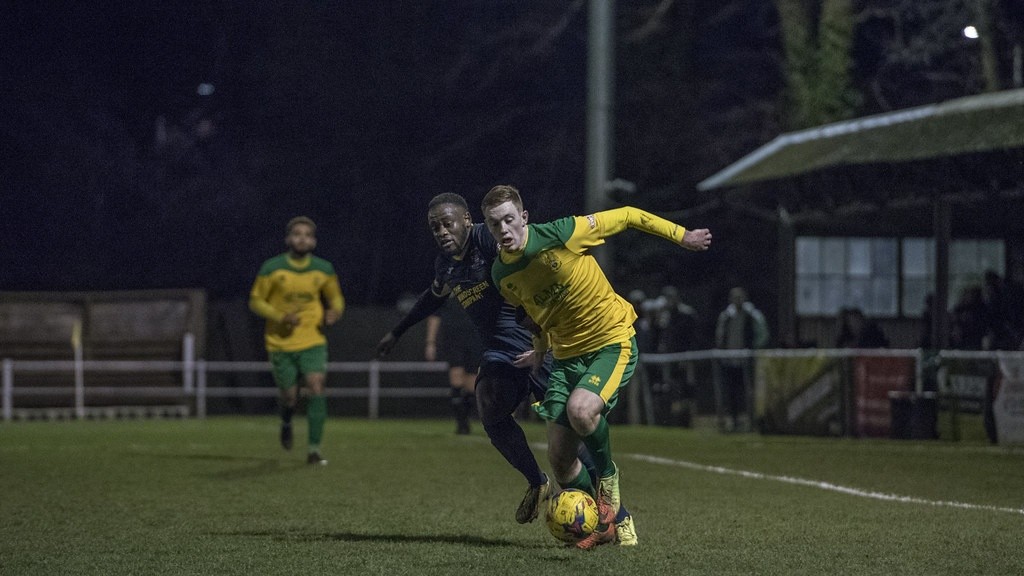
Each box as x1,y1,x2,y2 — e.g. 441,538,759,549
251,217,343,465
374,192,638,549
629,284,769,431
837,308,888,348
480,185,712,547
950,283,997,351
425,302,484,435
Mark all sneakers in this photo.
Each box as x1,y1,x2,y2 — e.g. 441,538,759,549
576,522,615,550
280,420,294,450
595,460,621,525
515,472,555,524
306,451,321,465
614,515,639,547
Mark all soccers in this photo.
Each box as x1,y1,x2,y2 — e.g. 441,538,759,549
545,486,598,541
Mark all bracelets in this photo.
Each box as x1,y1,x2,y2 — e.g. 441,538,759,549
426,338,436,345
